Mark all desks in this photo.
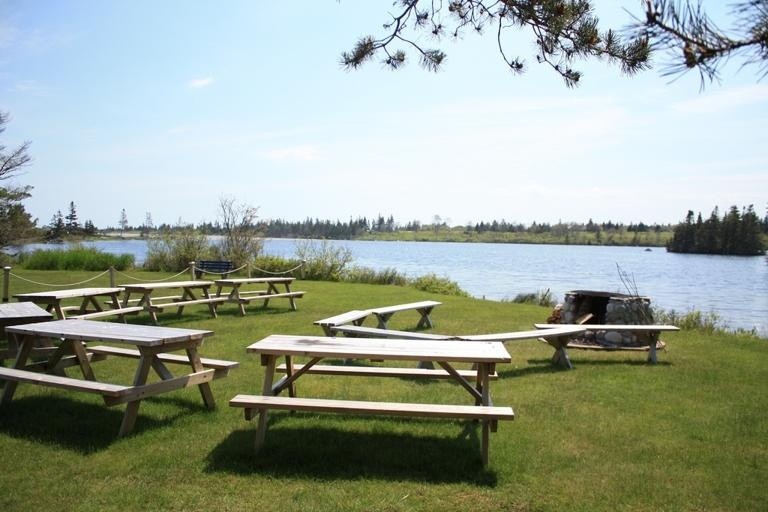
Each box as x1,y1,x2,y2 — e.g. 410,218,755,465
0,302,53,320
214,277,297,316
1,319,215,447
117,280,218,326
13,287,127,323
246,333,511,469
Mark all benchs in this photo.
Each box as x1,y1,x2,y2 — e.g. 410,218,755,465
201,290,267,298
228,393,513,431
195,259,235,278
66,306,144,320
237,291,307,301
145,296,229,307
49,306,80,313
276,364,499,386
86,344,238,373
0,366,134,399
102,296,183,305
